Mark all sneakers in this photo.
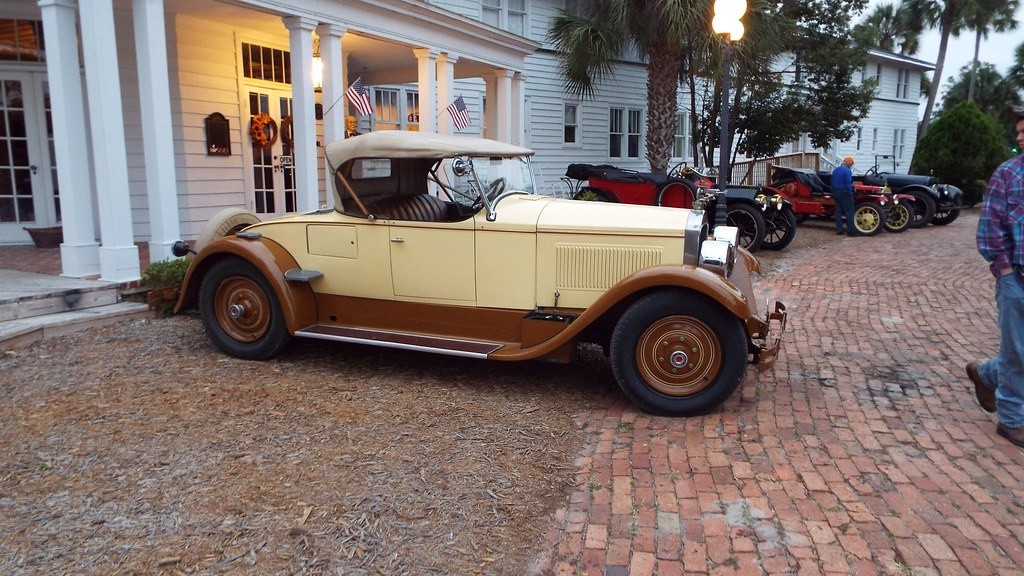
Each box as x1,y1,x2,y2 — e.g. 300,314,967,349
966,361,997,412
997,421,1024,448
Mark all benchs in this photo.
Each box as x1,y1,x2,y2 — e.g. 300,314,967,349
345,193,449,222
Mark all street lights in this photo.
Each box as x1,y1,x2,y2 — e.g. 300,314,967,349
711,0,747,226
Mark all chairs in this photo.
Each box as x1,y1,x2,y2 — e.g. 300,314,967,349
807,173,831,194
795,172,824,197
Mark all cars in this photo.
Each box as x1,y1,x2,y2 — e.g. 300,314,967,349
171,130,788,417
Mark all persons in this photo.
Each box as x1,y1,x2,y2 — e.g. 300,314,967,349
830,158,861,238
345,115,363,139
966,117,1024,447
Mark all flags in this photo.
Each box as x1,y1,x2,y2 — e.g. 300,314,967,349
345,76,374,117
446,96,472,131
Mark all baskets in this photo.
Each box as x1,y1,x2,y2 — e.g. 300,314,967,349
22,226,63,248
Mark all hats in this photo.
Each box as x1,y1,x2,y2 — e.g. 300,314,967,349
844,157,854,165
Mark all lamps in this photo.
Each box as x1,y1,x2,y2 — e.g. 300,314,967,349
311,39,323,89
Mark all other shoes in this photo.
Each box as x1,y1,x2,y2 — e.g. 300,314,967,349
837,231,844,235
848,232,860,237
